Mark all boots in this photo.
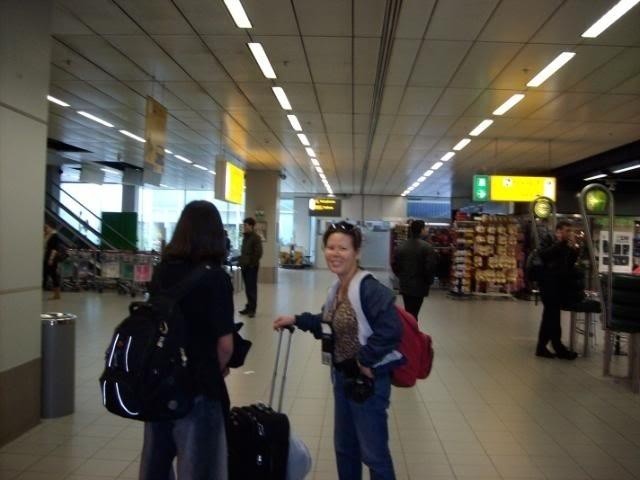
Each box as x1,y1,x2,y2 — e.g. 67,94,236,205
49,288,60,299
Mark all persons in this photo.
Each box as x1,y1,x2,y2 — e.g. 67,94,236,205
235,217,263,318
390,219,439,322
271,220,405,480
44,220,62,301
138,199,235,479
535,220,581,359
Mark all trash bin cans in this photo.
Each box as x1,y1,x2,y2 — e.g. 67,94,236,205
40,310,77,419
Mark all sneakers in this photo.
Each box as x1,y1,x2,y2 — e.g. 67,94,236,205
239,308,256,317
535,347,578,359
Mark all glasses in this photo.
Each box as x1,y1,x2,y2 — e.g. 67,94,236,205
328,223,354,232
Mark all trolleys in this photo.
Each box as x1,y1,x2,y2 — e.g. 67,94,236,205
43,249,160,299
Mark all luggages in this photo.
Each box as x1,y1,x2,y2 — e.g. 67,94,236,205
226,322,295,479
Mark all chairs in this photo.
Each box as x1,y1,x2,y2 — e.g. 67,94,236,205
560,272,599,357
597,271,640,390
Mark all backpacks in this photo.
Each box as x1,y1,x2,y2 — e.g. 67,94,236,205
99,263,210,423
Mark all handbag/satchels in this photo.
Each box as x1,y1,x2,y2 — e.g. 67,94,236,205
57,242,66,259
383,304,433,388
554,267,586,303
526,249,545,281
343,364,374,402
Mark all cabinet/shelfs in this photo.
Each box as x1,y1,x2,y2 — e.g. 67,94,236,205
391,214,594,299
